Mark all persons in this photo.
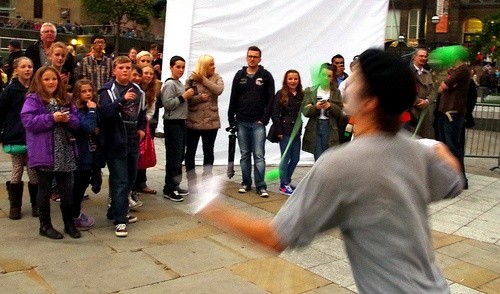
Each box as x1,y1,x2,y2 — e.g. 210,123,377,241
185,55,224,180
197,47,464,294
229,46,275,196
129,48,137,65
98,55,147,237
48,42,70,84
72,78,100,230
331,54,352,144
25,22,74,86
406,47,477,189
15,16,72,33
0,55,38,220
21,66,81,239
270,70,303,195
477,63,500,103
302,63,343,161
108,51,161,208
81,35,114,91
150,44,162,80
2,39,23,81
161,56,194,201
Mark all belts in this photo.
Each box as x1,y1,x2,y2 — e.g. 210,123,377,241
283,120,295,123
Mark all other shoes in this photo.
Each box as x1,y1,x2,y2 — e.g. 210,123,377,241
139,186,157,194
128,190,144,207
83,193,89,200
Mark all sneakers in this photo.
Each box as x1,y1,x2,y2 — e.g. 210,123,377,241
114,223,128,236
163,190,184,201
258,189,269,197
178,189,188,195
126,215,137,223
279,184,293,195
238,185,251,192
49,192,61,201
73,213,94,231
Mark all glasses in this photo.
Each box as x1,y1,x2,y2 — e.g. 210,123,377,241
93,41,105,44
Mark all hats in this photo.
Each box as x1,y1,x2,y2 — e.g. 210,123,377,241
136,51,153,59
8,39,21,48
385,40,416,57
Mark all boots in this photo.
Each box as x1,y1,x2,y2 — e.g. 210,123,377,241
6,181,23,220
64,213,80,238
28,182,39,217
39,203,63,239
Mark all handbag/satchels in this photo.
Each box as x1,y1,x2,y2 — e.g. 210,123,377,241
138,120,157,170
267,125,280,143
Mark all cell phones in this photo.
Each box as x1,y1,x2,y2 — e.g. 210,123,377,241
63,112,70,115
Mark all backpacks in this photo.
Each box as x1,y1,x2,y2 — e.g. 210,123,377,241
462,78,476,128
